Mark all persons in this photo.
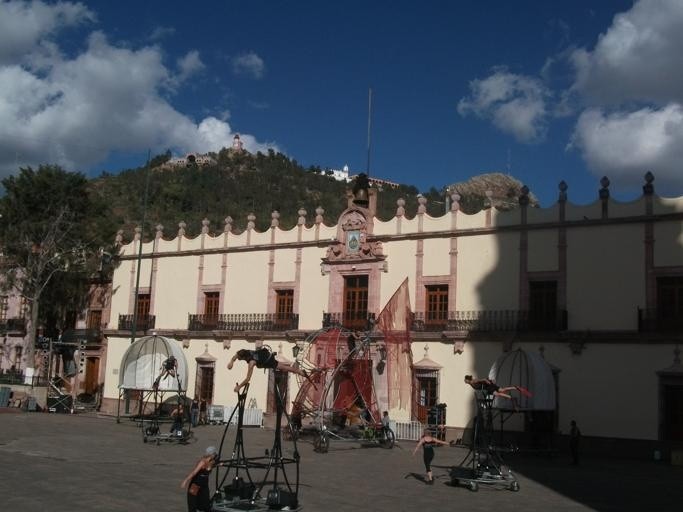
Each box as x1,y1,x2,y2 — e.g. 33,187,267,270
226,348,309,392
410,428,451,485
159,355,178,378
178,445,224,512
197,395,209,425
189,396,200,428
380,411,391,444
169,403,183,433
566,419,582,467
464,374,522,400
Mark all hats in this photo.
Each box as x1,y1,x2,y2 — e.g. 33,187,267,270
205,447,217,456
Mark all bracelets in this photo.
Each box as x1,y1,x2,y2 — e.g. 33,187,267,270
184,478,190,483
